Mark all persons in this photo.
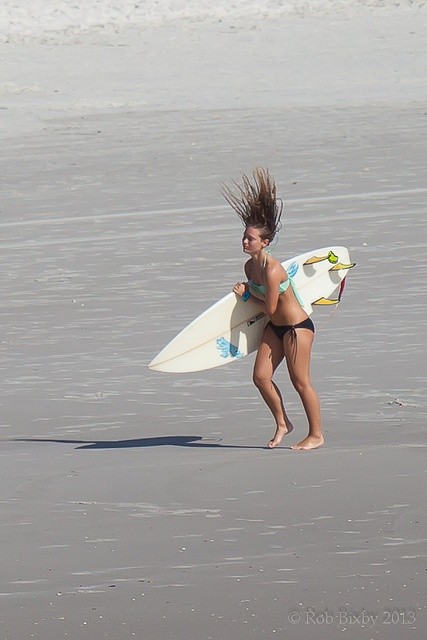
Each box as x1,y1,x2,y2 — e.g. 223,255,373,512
216,165,324,450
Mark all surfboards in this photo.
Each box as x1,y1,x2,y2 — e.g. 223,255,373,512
147,246,356,374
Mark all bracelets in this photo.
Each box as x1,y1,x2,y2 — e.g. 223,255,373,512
242,291,250,303
